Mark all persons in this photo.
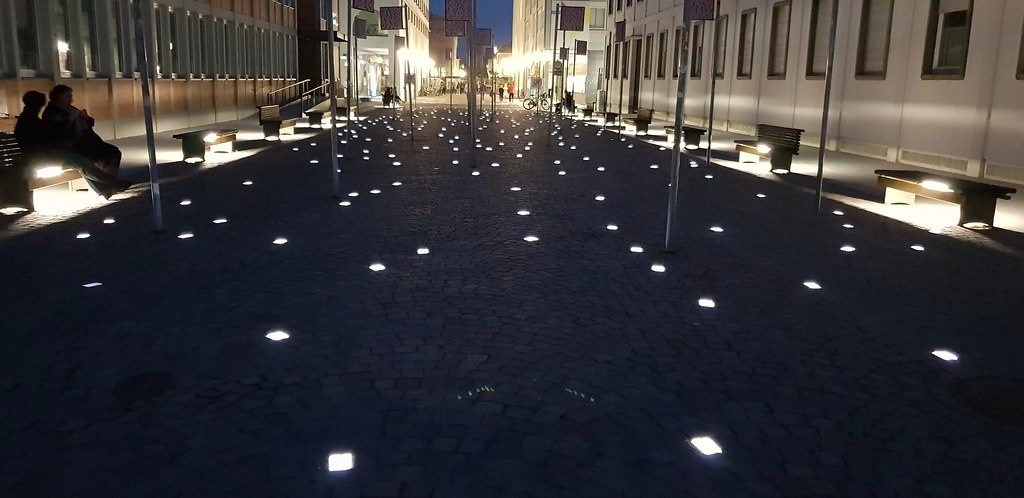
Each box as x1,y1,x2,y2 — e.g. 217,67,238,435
508,83,515,102
464,82,467,93
14,84,132,200
385,87,404,104
499,85,504,100
456,83,460,94
441,82,446,94
565,90,572,108
481,82,486,100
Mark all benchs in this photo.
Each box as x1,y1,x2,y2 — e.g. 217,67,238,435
874,170,1017,227
382,98,399,108
575,108,594,121
664,126,707,148
304,110,331,129
622,107,655,137
0,132,90,211
172,128,240,162
734,124,805,173
336,105,357,119
595,111,619,129
256,105,299,140
